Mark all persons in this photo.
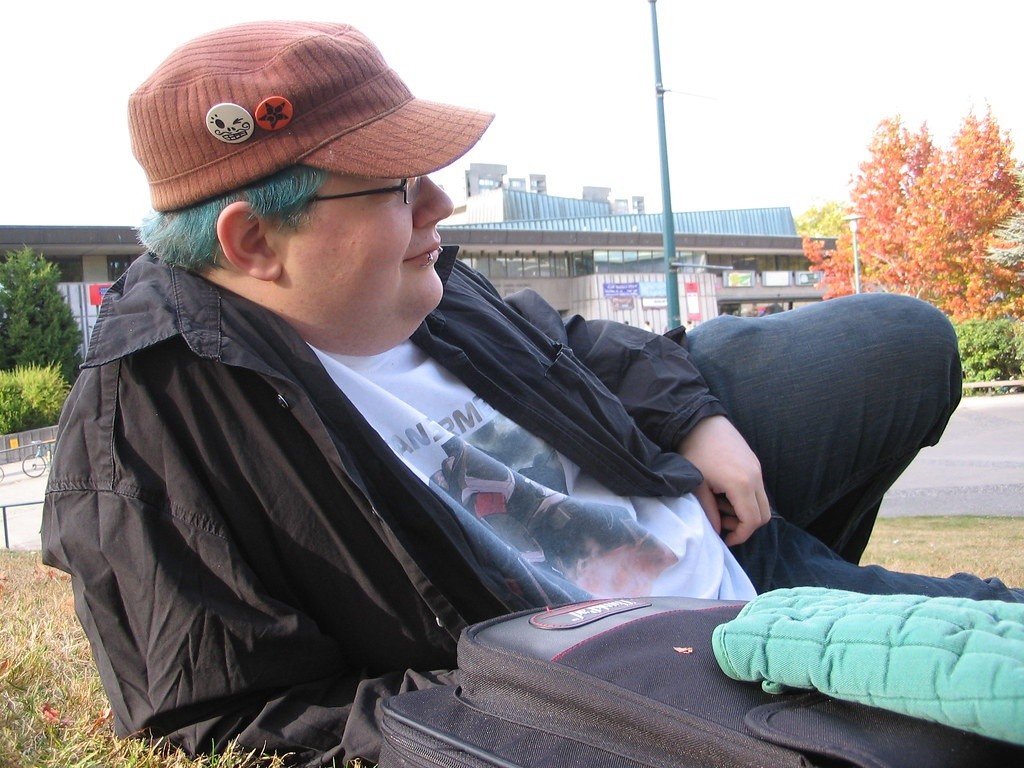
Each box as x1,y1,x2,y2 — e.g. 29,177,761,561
41,20,1023,768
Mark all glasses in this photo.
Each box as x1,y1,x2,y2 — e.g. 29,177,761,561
309,176,420,204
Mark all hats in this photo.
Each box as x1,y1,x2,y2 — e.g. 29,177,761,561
128,21,496,212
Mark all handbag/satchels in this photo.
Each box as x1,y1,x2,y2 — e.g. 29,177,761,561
376,596,1024,768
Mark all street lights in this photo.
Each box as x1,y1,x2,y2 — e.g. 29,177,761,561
844,209,867,293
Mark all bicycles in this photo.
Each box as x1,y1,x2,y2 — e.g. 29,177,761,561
21,440,58,478
